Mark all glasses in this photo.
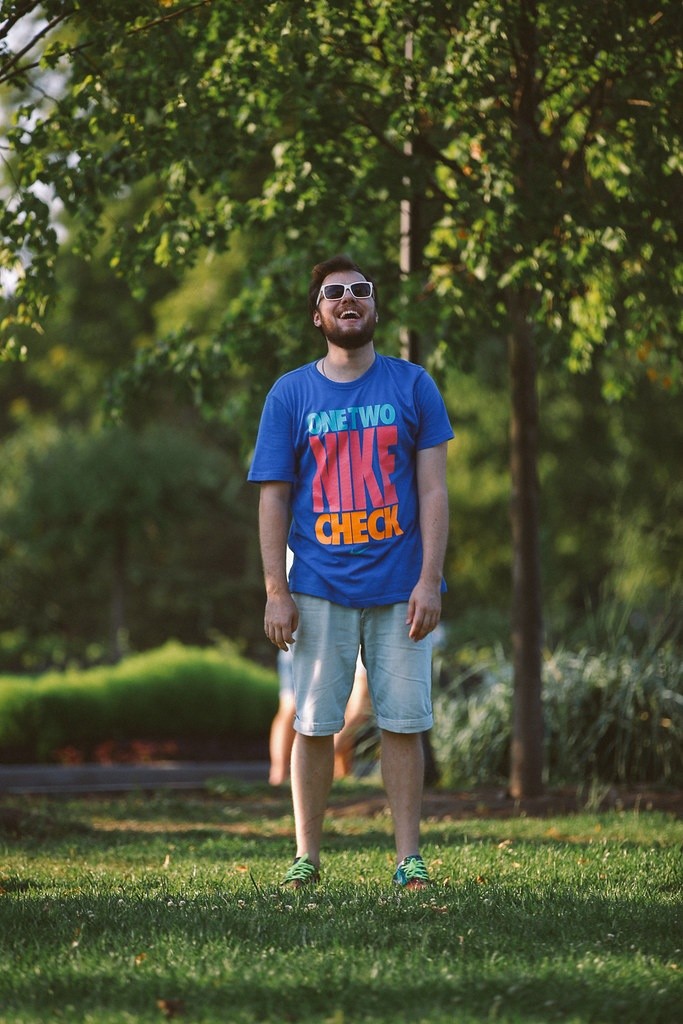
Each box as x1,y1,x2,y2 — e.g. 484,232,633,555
315,281,376,306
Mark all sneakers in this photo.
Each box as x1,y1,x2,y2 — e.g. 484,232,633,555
390,855,429,892
278,857,322,892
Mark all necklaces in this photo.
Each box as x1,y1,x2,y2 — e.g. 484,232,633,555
322,358,327,377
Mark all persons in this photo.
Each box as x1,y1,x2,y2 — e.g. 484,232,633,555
246,259,454,890
268,544,298,787
330,646,370,776
